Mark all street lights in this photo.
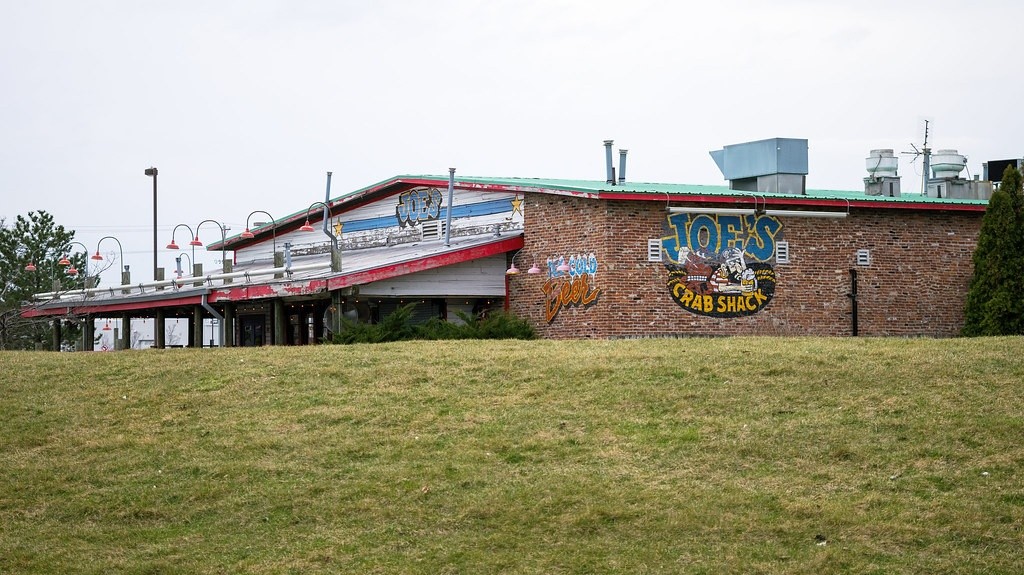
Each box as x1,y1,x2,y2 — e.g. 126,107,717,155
57,242,89,288
240,210,277,266
101,318,120,334
165,224,196,277
142,167,159,283
91,235,123,284
24,248,54,295
188,217,225,285
300,201,333,256
